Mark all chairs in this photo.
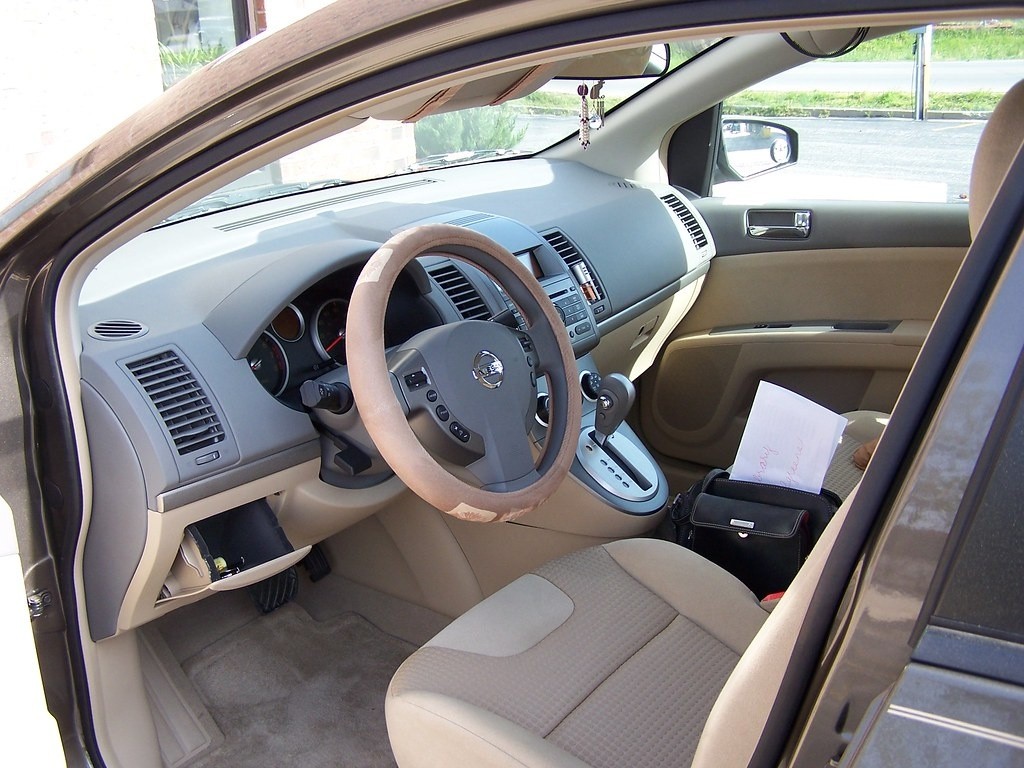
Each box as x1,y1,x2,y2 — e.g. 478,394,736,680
725,411,892,505
384,78,1024,768
